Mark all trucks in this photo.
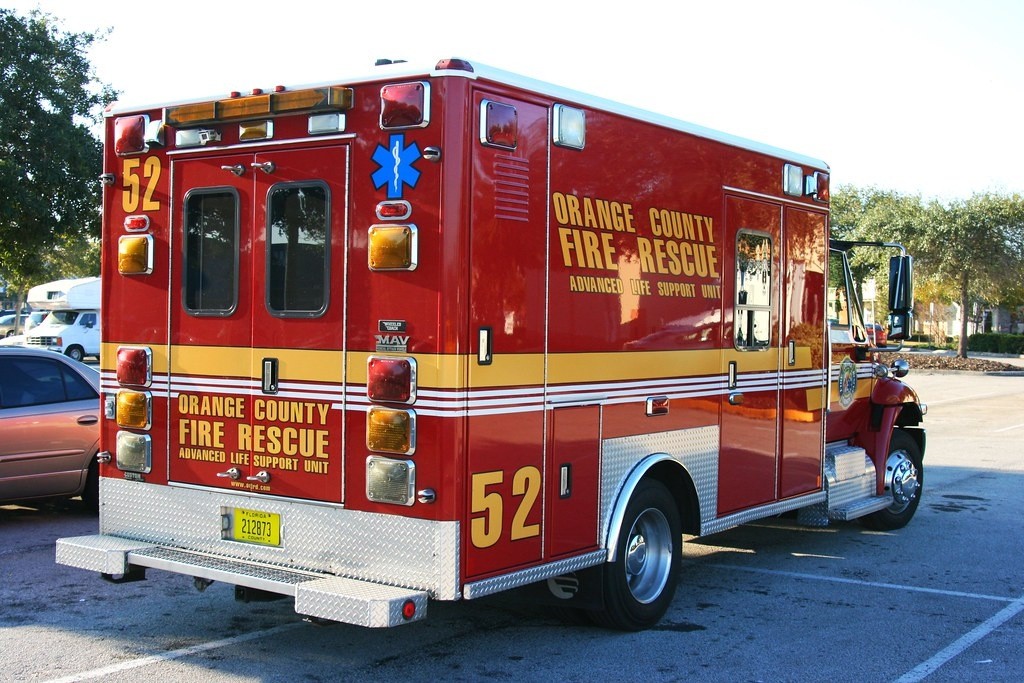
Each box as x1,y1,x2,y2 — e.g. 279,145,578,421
23,276,102,362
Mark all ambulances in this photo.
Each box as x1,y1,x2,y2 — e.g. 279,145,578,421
49,53,933,632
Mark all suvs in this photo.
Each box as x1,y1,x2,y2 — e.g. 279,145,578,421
23,312,50,332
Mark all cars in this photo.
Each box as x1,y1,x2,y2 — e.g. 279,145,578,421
864,323,888,349
0,314,29,339
0,343,104,511
